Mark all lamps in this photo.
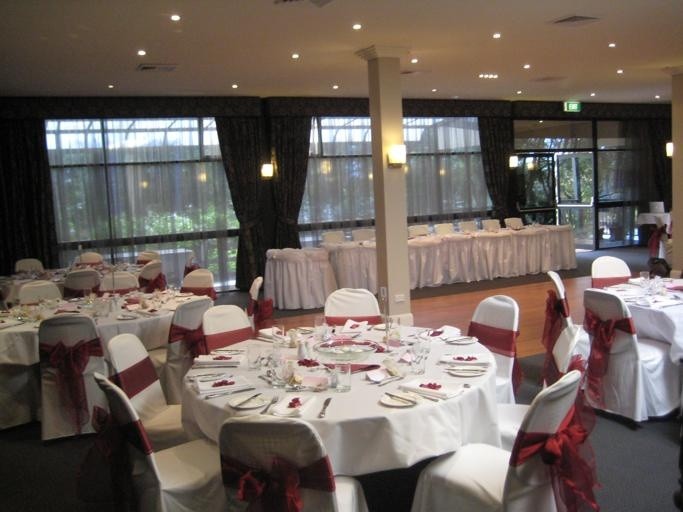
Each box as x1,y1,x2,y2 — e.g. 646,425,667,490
260,162,273,178
665,142,673,158
388,144,407,170
507,155,518,170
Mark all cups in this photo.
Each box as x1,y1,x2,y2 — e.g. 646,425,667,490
271,324,284,352
247,343,261,370
384,316,400,350
655,275,663,287
639,271,649,286
409,340,431,375
334,362,351,392
313,315,325,339
327,375,336,388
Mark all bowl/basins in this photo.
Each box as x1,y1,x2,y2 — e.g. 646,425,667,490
312,339,378,365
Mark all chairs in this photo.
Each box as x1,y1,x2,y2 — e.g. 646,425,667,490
536,270,598,387
322,217,524,245
498,323,591,452
324,289,382,328
93,371,228,511
466,295,518,404
590,218,681,289
0,249,265,335
218,415,367,512
582,289,680,430
146,296,214,405
201,305,256,356
107,333,207,450
39,313,109,441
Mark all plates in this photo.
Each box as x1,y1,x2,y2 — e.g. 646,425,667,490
272,406,305,416
446,336,477,345
450,365,484,377
295,326,314,334
228,393,268,410
381,394,417,406
419,329,440,340
373,323,397,331
635,298,650,306
187,369,233,382
1,287,193,328
212,347,242,355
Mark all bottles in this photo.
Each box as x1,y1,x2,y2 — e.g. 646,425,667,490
288,329,296,347
296,339,307,359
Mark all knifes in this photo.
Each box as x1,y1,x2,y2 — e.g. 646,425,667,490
204,387,256,399
444,368,486,372
384,391,416,404
319,397,331,416
235,392,261,407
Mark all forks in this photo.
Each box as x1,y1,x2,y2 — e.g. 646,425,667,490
260,391,279,414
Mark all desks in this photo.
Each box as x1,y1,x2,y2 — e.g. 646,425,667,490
603,276,683,365
263,224,575,309
184,324,497,511
1,293,214,429
